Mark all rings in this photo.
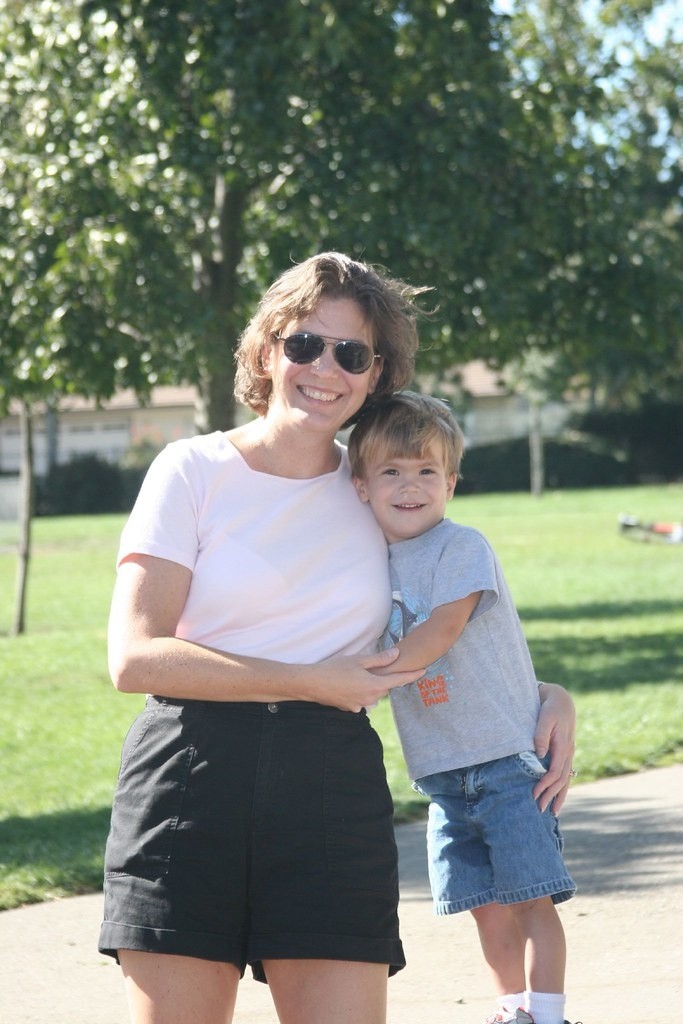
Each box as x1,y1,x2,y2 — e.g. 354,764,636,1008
570,768,578,776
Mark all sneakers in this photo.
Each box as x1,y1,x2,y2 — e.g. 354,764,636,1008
485,1005,583,1024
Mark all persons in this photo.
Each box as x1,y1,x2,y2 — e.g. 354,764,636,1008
347,391,580,1023
98,250,575,1024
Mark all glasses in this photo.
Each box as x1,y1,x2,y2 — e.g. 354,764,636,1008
271,331,382,375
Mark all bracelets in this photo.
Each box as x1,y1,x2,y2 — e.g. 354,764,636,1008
537,682,543,687
